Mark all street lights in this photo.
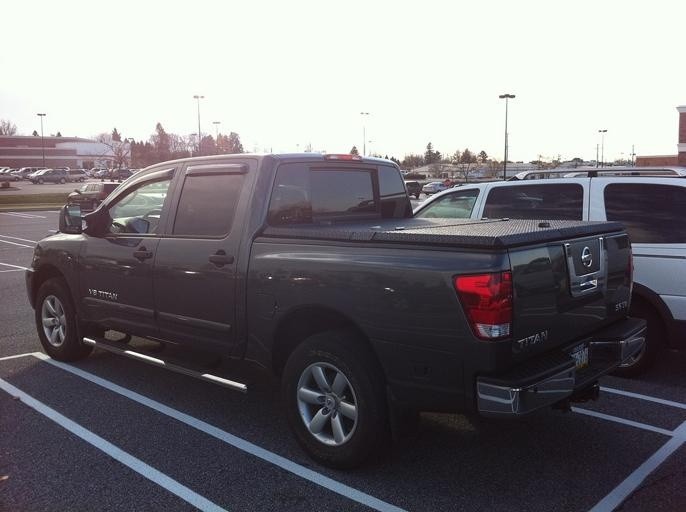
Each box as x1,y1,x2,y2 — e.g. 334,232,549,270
498,93,514,180
193,95,204,156
37,113,46,168
597,129,606,168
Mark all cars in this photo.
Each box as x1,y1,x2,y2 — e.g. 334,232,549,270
421,181,448,195
0,166,141,184
404,181,421,199
67,182,164,219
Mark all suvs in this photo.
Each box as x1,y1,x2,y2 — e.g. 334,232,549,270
411,166,685,378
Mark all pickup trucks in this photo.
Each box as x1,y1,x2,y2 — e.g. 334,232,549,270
24,152,649,470
